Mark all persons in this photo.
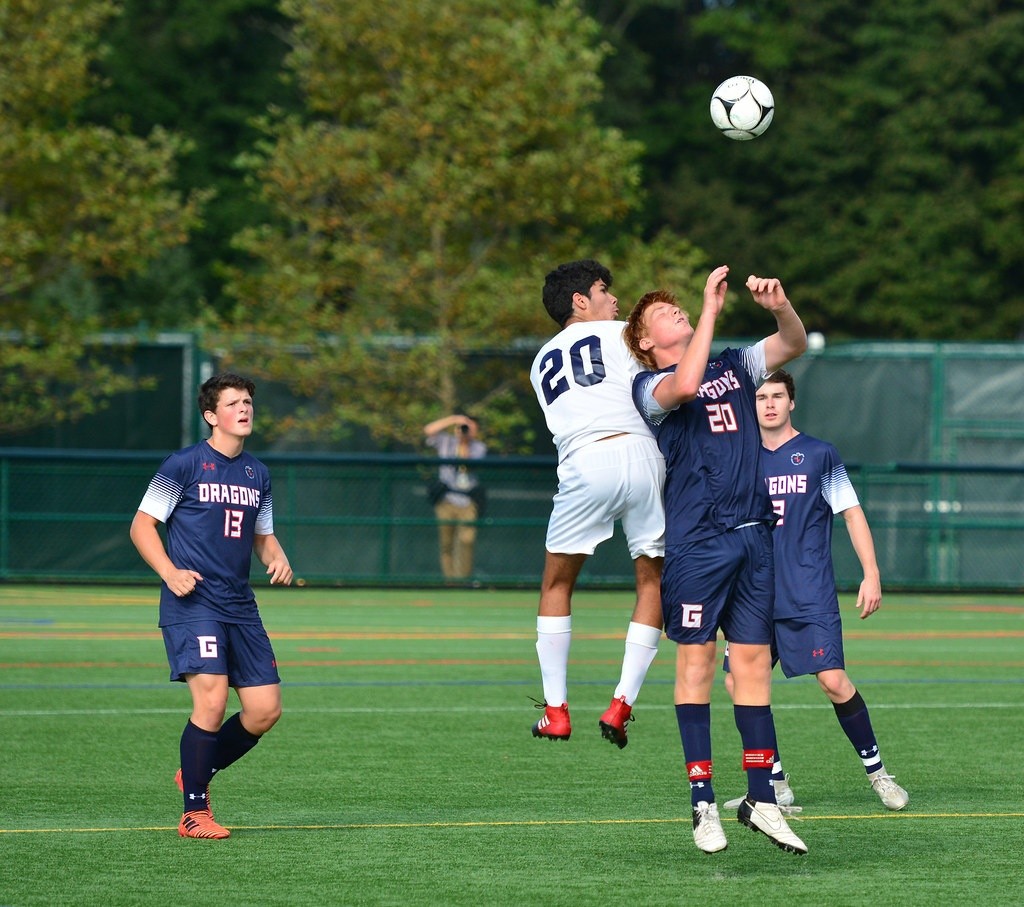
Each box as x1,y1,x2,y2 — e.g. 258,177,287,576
530,259,668,748
129,374,294,839
423,412,489,587
621,265,808,853
725,369,909,813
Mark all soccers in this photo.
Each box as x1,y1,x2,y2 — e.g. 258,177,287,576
709,74,776,142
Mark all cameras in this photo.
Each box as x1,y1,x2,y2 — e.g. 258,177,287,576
461,424,467,434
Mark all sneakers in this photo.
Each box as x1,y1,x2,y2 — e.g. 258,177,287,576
737,793,808,856
598,695,635,750
178,810,230,839
724,773,795,810
526,695,572,741
690,801,727,853
866,764,909,811
175,767,216,823
